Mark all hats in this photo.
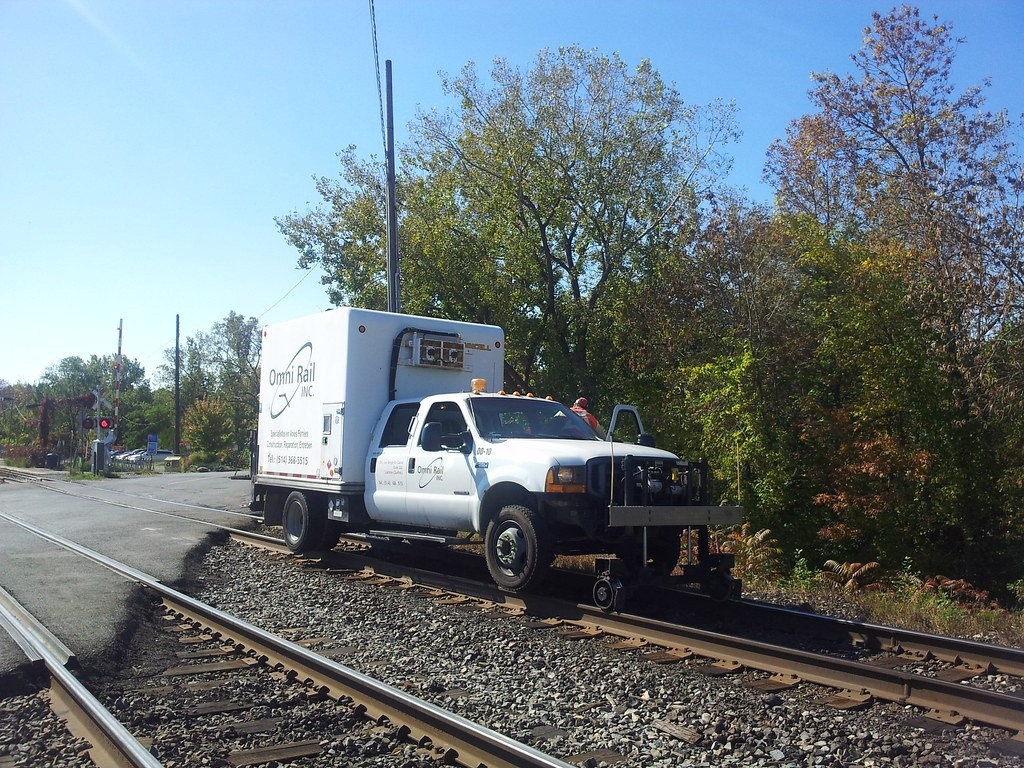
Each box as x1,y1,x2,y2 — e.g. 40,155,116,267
575,398,588,410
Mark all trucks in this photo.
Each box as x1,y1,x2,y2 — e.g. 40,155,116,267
252,306,688,592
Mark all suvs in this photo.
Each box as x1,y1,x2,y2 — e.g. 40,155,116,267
109,447,175,463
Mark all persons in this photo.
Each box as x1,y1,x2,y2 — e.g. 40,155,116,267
554,397,606,439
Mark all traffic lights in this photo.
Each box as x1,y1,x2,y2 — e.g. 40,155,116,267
83,417,94,429
98,418,110,429
